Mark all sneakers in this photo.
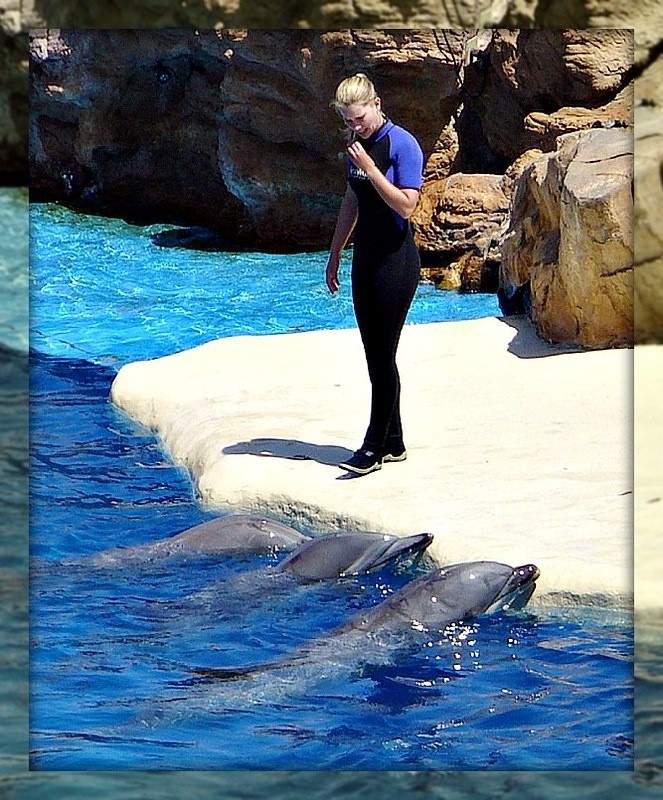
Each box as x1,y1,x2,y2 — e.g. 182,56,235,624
339,447,382,475
381,442,407,464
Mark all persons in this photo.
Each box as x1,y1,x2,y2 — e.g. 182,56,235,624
326,72,424,474
